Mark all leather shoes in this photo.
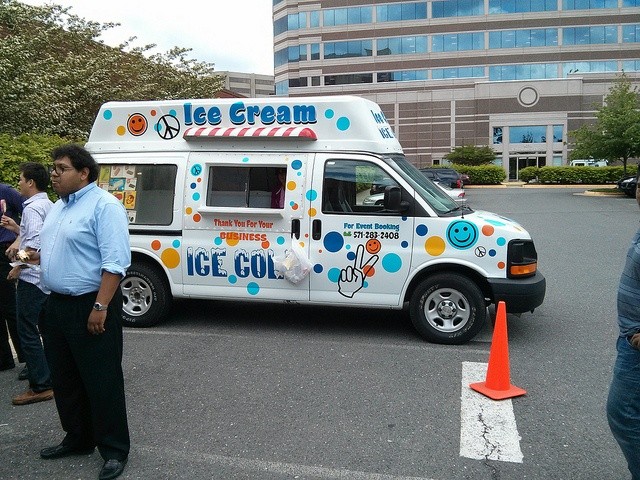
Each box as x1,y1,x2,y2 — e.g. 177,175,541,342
39,440,98,459
12,389,53,406
97,457,128,479
17,362,27,381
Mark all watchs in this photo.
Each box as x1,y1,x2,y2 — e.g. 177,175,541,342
93,302,109,311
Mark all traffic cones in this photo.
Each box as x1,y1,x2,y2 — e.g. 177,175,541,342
469,302,526,400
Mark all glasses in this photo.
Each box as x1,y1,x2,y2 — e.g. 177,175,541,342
48,162,78,176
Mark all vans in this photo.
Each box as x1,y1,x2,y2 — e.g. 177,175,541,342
82,96,546,344
570,159,607,169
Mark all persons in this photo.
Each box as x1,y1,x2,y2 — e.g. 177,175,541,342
0,183,31,381
272,168,287,208
15,145,133,480
605,165,640,479
0,162,55,406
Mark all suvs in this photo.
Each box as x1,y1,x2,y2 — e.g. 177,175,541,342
370,169,462,193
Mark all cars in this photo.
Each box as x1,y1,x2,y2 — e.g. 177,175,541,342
618,178,637,193
363,182,467,206
